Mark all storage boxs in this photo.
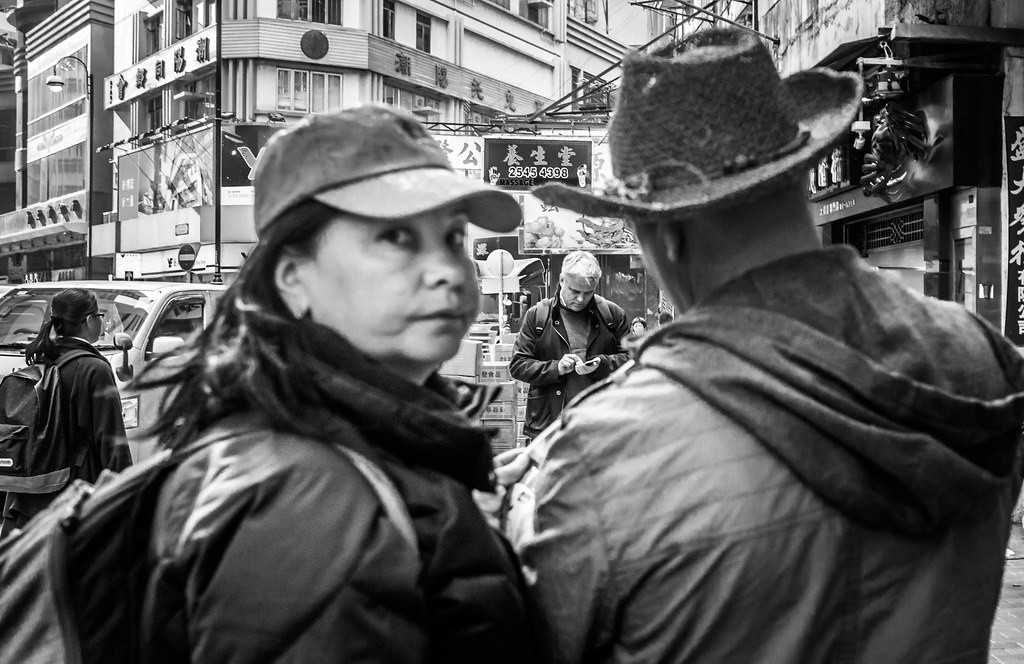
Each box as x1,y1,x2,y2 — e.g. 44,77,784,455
437,333,531,456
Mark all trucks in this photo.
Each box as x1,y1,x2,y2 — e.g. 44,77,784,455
0,281,232,465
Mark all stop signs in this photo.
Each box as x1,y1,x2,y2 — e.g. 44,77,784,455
178,244,196,270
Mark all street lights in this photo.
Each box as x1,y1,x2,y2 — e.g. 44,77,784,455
46,55,94,281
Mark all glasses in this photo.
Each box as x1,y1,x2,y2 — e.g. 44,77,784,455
82,313,105,323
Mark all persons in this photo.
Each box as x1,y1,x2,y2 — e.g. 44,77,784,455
519,26,1024,664
630,317,647,334
0,288,134,542
659,313,673,325
509,250,631,440
93,102,565,664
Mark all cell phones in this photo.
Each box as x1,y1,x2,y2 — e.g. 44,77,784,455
585,360,598,366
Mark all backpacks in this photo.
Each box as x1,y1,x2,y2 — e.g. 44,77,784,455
0,425,418,664
0,350,109,494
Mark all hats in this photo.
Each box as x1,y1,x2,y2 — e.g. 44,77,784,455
531,28,865,218
254,102,522,235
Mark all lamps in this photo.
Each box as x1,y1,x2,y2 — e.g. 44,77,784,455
268,112,285,122
138,130,172,140
172,116,203,128
109,139,140,147
126,135,154,143
95,143,126,152
154,124,189,136
214,112,241,123
